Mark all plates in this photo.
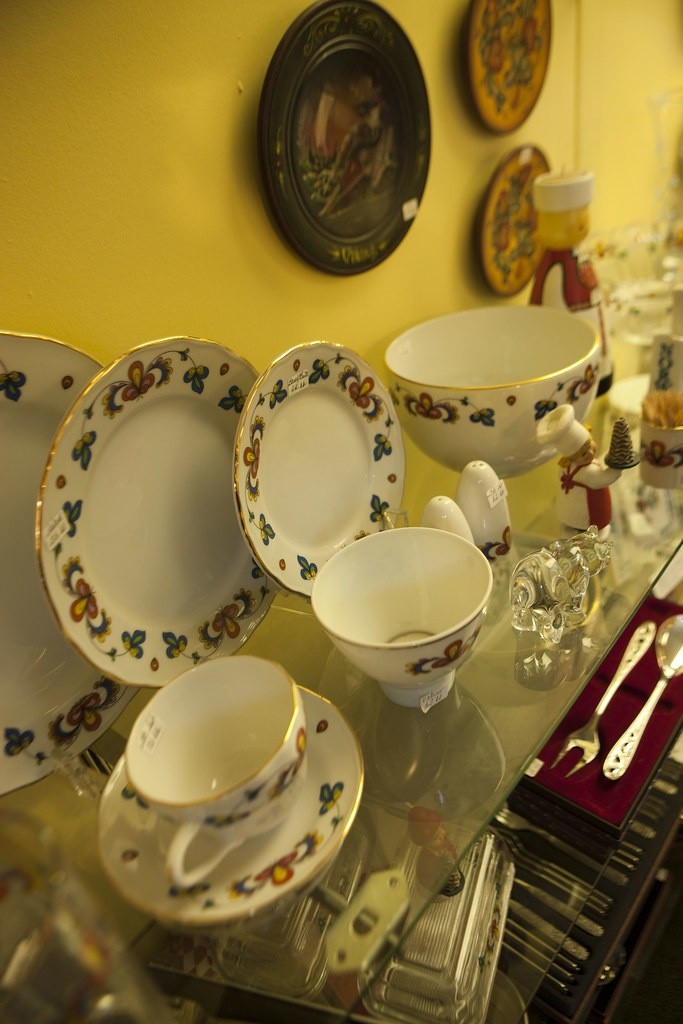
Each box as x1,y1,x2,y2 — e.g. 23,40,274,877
478,143,550,297
0,330,141,796
98,687,365,925
232,341,405,598
33,336,281,690
255,0,432,276
467,0,554,136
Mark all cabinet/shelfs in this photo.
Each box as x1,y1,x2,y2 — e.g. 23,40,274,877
0,475,683,1024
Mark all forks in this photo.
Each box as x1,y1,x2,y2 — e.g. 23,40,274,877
549,621,656,778
494,808,630,917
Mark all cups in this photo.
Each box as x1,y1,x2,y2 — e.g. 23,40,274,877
126,655,309,891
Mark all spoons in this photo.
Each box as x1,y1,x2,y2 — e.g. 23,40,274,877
603,614,683,780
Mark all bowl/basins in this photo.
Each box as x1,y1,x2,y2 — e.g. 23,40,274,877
382,305,602,479
310,528,494,709
